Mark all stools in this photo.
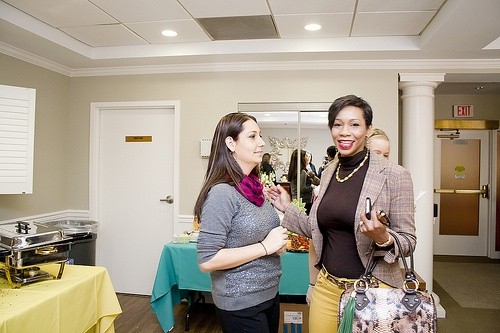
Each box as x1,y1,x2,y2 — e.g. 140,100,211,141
401,268,426,291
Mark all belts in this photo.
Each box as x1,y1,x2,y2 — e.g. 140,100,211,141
321,267,354,290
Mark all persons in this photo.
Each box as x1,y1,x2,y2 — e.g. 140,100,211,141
366,128,391,160
265,94,418,333
259,153,277,186
324,145,337,169
304,152,318,177
287,149,316,217
318,154,329,179
195,112,289,333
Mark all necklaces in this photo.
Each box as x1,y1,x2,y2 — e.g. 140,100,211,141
335,147,369,183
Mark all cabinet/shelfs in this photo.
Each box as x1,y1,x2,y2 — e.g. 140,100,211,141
272,181,292,200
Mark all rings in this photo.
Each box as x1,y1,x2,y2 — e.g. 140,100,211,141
359,220,364,225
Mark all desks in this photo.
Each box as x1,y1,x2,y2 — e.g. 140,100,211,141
163,241,310,333
0,262,123,333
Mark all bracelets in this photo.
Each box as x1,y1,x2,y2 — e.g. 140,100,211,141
375,233,394,248
260,242,268,256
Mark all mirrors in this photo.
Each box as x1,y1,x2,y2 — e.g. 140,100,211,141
298,110,339,217
238,110,298,200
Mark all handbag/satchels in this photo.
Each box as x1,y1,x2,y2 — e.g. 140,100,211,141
337,231,437,333
